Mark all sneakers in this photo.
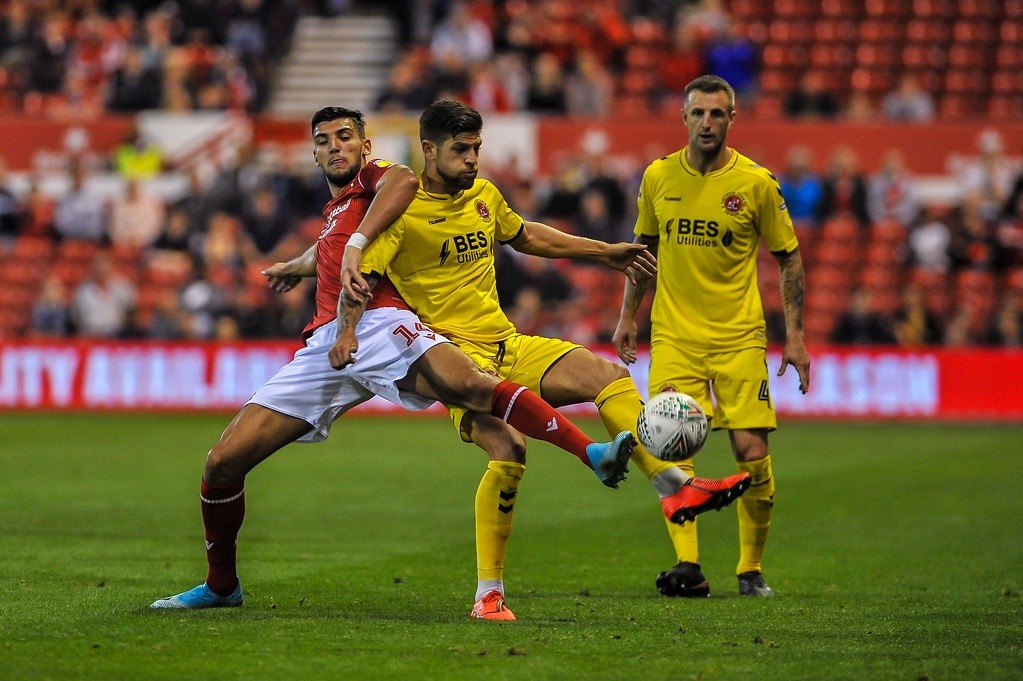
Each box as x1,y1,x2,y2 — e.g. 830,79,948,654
149,576,244,610
736,570,773,596
655,561,711,598
660,472,752,524
471,590,517,621
585,431,639,489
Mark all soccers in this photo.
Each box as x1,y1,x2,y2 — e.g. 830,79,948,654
637,391,708,461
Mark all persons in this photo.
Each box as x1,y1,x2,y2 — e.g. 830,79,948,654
327,98,752,624
149,107,639,608
610,74,812,601
1,1,1023,351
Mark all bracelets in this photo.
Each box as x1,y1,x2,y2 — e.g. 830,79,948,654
345,232,370,251
